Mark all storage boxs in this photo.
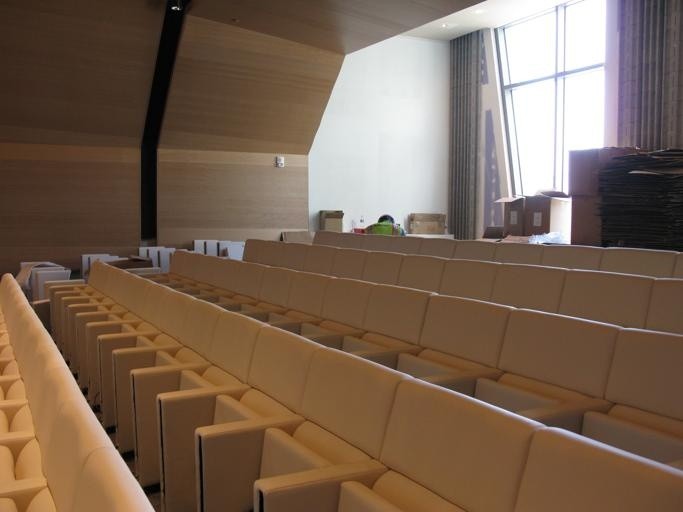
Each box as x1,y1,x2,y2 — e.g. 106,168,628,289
408,213,446,235
319,209,344,233
476,146,649,246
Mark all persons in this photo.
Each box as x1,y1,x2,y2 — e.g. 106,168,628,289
378,215,394,223
